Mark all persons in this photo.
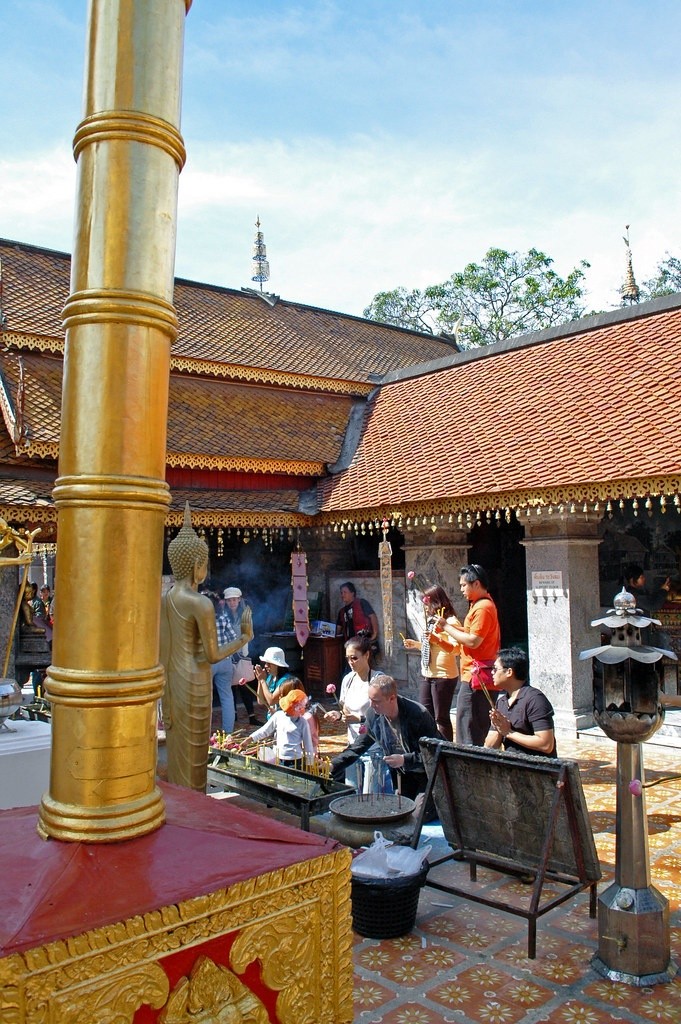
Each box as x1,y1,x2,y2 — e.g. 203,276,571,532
224,587,265,726
21,580,46,633
626,567,671,644
484,648,557,759
437,563,501,746
269,679,318,754
402,584,462,741
322,636,393,794
337,582,379,640
237,690,314,770
313,675,447,798
159,500,254,793
200,592,238,733
253,647,294,718
30,582,54,703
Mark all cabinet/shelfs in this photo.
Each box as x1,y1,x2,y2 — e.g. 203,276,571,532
259,636,343,700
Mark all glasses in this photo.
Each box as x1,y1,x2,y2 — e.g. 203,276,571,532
491,665,507,674
345,654,363,662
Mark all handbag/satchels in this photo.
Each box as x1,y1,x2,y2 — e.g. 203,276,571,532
347,829,432,876
231,657,256,686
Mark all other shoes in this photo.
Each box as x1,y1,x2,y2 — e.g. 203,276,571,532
249,716,266,726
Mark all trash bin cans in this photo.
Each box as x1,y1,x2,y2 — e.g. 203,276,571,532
347,845,430,941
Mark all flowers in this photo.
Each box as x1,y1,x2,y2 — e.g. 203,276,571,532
238,678,270,709
629,775,681,796
358,725,388,755
408,570,436,615
209,728,275,755
325,684,344,714
470,666,494,709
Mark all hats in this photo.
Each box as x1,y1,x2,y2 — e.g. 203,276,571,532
279,688,309,716
259,647,289,667
223,587,242,599
